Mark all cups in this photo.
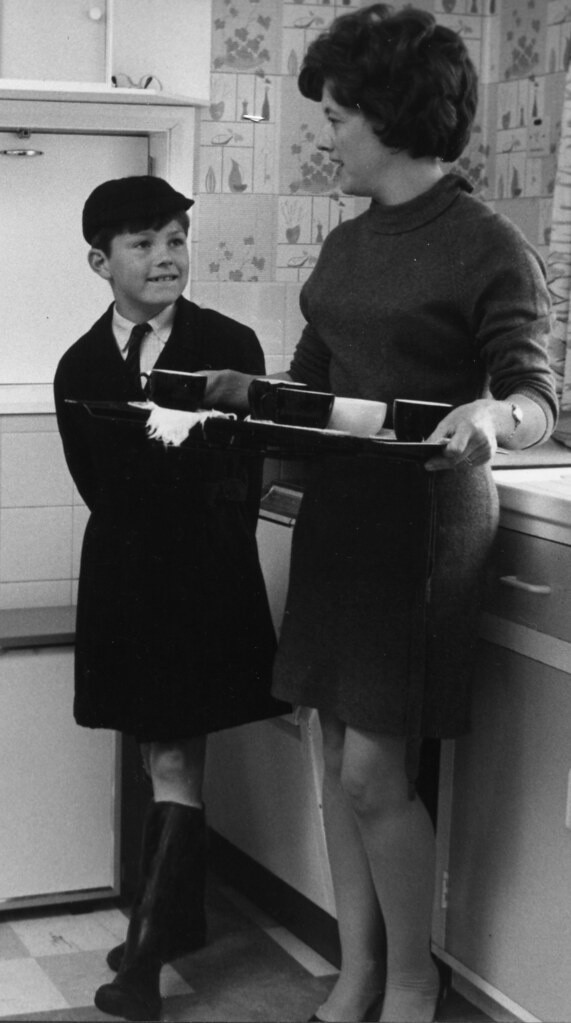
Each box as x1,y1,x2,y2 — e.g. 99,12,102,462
276,387,335,429
393,398,453,444
327,396,387,436
130,368,208,412
248,378,308,422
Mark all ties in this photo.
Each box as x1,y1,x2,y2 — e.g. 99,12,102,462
122,323,153,394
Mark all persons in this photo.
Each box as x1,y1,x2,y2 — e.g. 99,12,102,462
200,6,560,1023
54,175,278,1022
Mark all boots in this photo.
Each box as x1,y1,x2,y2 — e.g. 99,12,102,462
94,802,205,1021
106,799,208,972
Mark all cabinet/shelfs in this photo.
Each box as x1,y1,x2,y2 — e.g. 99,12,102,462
0,455,571,1023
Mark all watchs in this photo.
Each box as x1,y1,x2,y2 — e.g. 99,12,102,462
500,400,523,448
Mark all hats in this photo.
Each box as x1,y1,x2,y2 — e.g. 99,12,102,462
82,174,194,244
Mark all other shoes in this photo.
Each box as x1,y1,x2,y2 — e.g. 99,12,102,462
307,992,385,1023
377,970,444,1023
